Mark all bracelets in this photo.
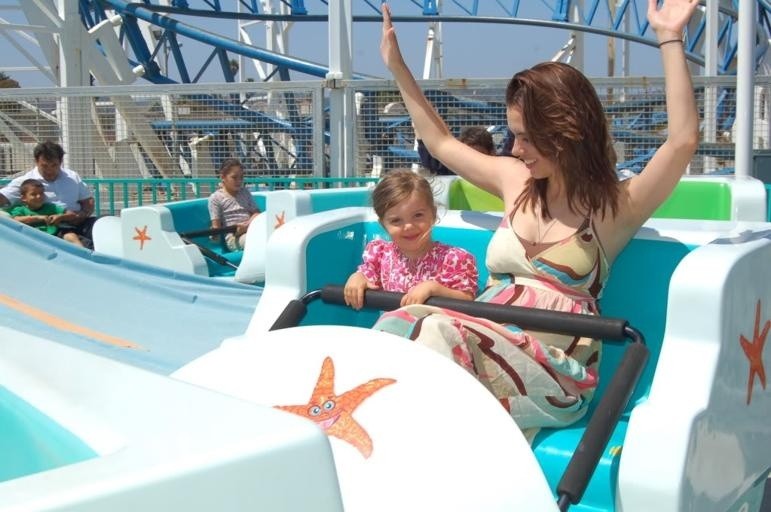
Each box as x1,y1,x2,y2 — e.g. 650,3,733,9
657,39,682,47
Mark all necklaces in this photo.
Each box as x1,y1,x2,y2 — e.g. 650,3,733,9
535,209,561,243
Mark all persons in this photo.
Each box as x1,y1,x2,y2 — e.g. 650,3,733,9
370,0,701,436
411,122,506,179
7,178,83,248
343,161,478,316
0,139,97,252
206,157,261,253
